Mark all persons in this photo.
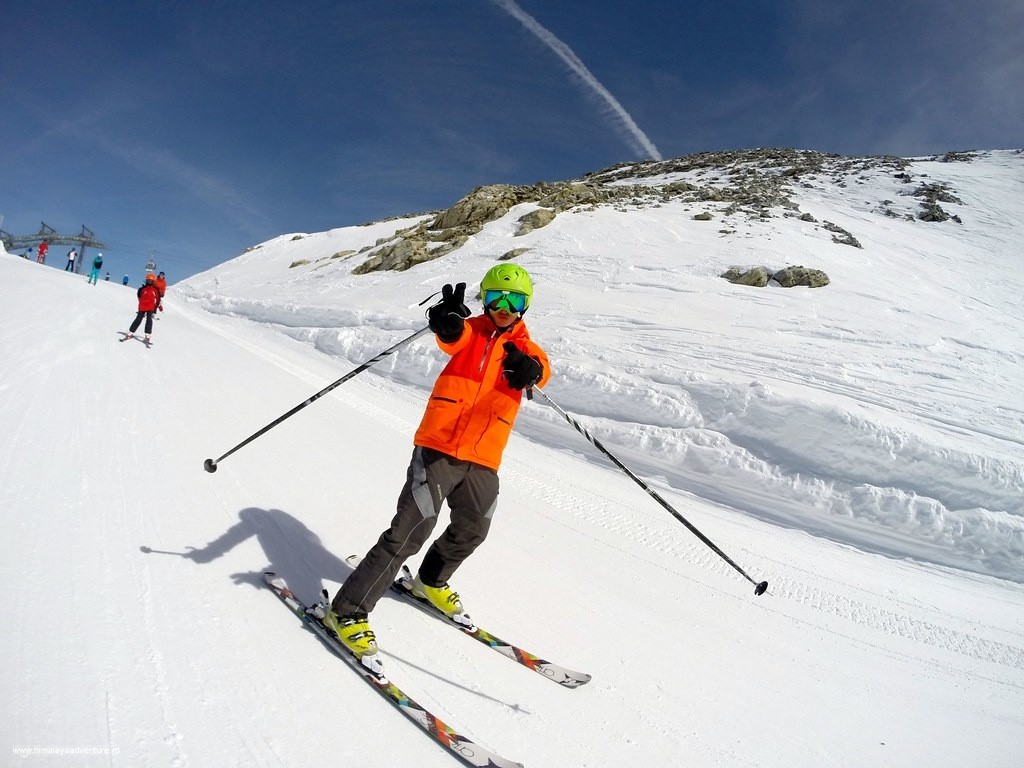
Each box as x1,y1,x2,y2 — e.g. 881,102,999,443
153,272,166,313
66,248,78,272
106,272,110,281
36,239,49,263
127,274,160,342
27,245,32,256
123,275,128,285
326,263,552,654
88,252,104,286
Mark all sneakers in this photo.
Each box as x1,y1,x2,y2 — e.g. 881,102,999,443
412,572,464,619
94,283,96,286
322,604,378,656
144,334,152,345
88,281,91,284
127,332,134,339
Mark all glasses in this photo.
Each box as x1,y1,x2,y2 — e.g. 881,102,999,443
483,290,527,312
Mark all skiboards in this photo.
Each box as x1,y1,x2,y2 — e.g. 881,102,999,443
119,332,153,348
265,555,594,766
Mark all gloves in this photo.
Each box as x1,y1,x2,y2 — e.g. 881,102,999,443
159,305,163,312
502,341,542,391
418,283,472,344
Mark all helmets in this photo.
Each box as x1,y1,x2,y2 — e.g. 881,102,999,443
107,272,110,275
145,273,156,281
480,263,534,315
98,253,103,256
125,274,128,276
